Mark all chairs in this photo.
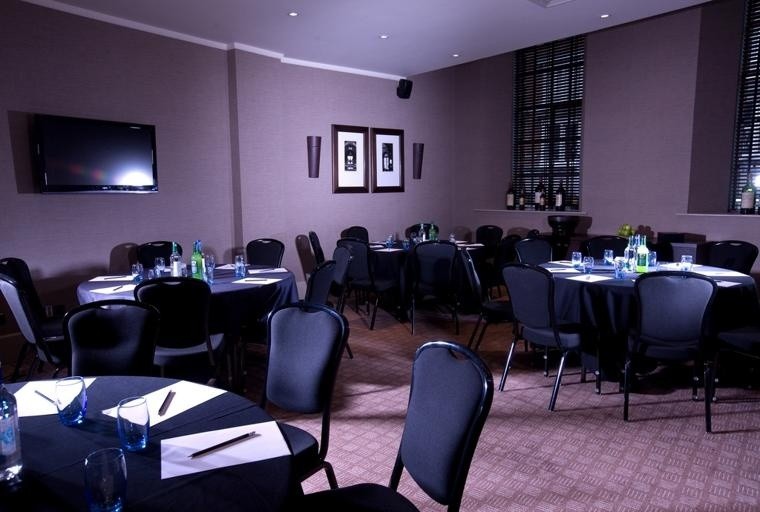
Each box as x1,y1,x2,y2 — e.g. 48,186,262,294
255,300,351,490
1,224,760,386
618,268,722,433
498,261,609,413
303,339,496,511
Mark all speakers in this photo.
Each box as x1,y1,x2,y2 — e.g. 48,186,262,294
397,79,412,99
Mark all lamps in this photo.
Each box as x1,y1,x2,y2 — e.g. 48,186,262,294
307,134,322,177
414,142,425,179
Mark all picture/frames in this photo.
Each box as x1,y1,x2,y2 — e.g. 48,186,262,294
372,127,405,193
330,124,372,193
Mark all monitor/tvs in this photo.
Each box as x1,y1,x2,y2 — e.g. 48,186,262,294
30,113,158,193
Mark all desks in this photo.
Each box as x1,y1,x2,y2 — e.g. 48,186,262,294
0,374,307,510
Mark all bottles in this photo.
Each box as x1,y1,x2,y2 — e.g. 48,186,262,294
348,147,354,169
740,174,757,215
418,224,426,242
555,183,566,212
519,189,526,211
0,383,23,482
190,239,206,280
429,224,436,241
169,241,188,277
505,184,516,210
534,180,546,211
383,151,393,170
624,234,649,273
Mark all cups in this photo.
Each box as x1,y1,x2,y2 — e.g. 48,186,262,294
132,263,144,285
448,232,456,243
614,256,626,280
603,250,613,265
116,396,150,452
205,254,216,274
572,251,582,267
82,447,128,512
648,251,657,267
402,238,410,249
411,232,417,238
147,257,166,281
583,256,594,269
235,255,247,278
385,230,393,250
680,255,693,272
55,376,88,427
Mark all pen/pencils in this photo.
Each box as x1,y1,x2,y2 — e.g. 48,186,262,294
713,280,722,283
104,276,126,280
158,389,172,413
35,390,55,403
550,268,565,271
559,261,572,264
113,285,123,291
260,268,275,273
245,279,266,282
187,431,256,458
676,263,680,266
